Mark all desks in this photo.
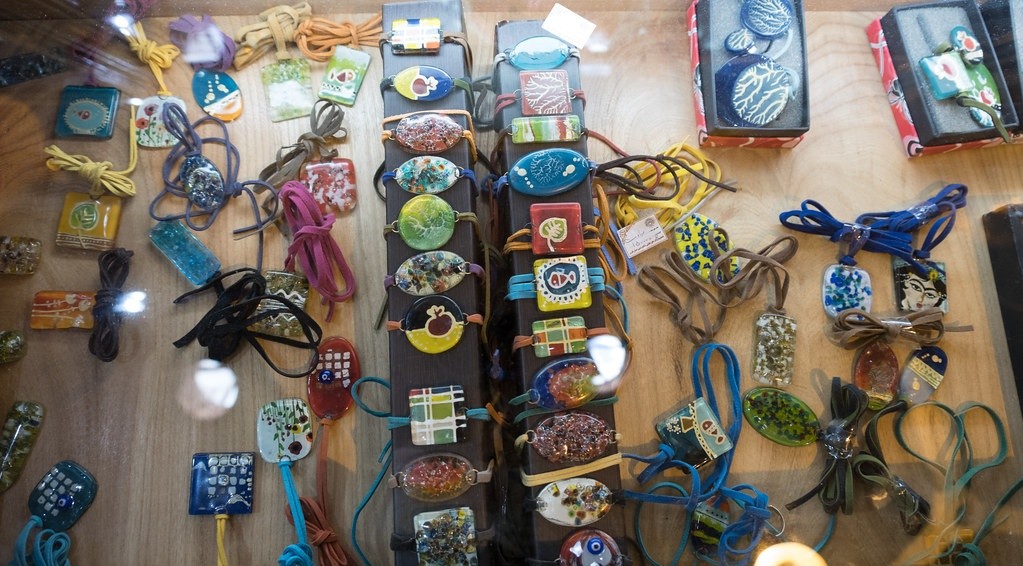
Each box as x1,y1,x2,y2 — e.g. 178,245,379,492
0,0,1023,566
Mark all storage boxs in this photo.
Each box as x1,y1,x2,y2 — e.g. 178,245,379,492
687,0,810,150
865,0,1023,160
